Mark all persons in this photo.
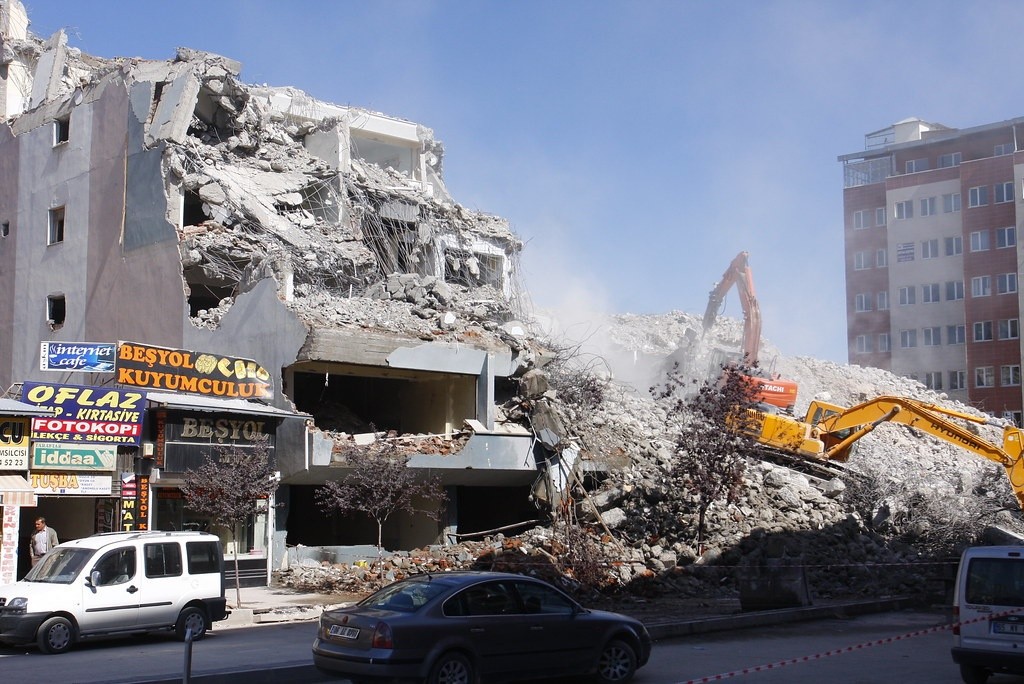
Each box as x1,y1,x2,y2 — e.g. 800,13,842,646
30,518,58,567
527,597,540,614
468,586,491,615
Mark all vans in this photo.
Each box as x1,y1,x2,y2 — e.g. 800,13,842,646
0,526,233,656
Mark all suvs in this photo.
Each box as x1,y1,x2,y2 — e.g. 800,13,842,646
950,542,1024,684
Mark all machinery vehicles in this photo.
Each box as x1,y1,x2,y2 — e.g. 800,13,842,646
661,250,799,411
724,391,1024,514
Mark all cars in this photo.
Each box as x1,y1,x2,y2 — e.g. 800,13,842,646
310,570,655,684
